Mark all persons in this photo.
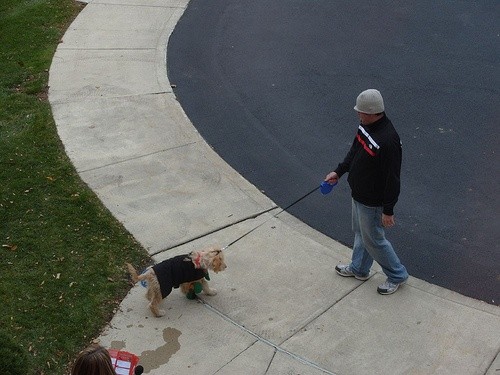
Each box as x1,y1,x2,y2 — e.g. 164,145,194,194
324,89,408,295
71,345,116,375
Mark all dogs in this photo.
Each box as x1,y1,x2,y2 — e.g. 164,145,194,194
125,248,227,318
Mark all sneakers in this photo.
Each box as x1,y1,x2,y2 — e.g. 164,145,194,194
377,274,408,295
335,264,371,281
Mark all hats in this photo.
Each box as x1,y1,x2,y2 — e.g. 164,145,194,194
354,88,384,115
